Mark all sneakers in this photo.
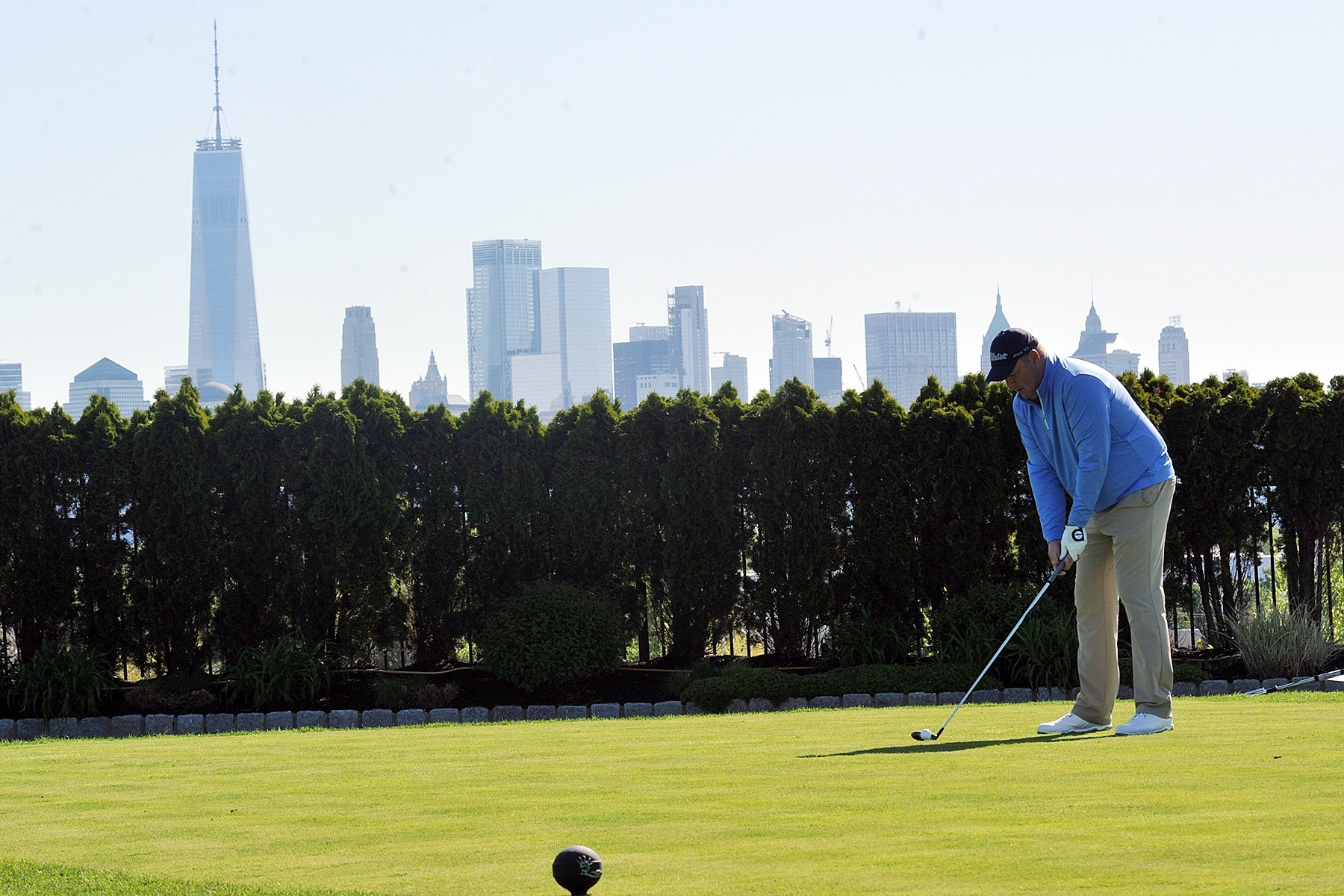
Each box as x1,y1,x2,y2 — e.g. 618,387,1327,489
1037,712,1114,734
1115,712,1175,736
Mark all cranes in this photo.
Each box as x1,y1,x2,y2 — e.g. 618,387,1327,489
824,315,833,357
853,364,865,389
713,352,730,355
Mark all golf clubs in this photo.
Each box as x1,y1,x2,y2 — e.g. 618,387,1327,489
911,557,1065,741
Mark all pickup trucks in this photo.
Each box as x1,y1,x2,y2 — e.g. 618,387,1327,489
1164,544,1282,585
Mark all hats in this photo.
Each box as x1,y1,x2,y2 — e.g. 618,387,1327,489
985,327,1038,382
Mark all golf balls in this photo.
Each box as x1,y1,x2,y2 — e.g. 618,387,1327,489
920,729,932,740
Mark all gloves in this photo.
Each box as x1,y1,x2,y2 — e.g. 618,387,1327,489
1059,524,1088,562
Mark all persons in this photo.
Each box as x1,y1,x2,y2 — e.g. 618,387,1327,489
985,327,1177,736
